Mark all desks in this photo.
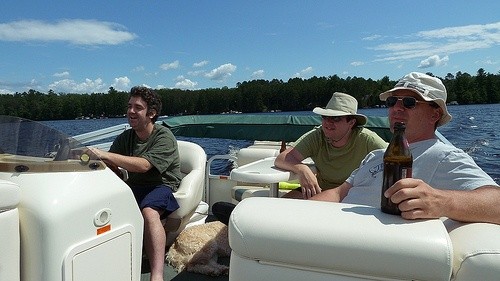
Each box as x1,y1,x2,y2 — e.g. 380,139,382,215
229,157,316,199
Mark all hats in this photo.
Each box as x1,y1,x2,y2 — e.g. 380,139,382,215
379,71,451,127
312,92,367,127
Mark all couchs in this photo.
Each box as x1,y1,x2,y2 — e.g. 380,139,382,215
228,196,452,281
237,140,315,167
440,216,500,281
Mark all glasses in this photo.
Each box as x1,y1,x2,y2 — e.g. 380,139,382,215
322,115,348,122
385,95,434,110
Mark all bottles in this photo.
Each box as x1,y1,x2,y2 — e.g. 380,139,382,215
381,122,413,216
280,137,286,154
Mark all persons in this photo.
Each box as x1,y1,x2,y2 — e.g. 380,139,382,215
274,92,388,199
302,73,500,223
79,86,182,281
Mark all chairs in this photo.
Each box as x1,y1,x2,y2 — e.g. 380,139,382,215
156,140,209,248
